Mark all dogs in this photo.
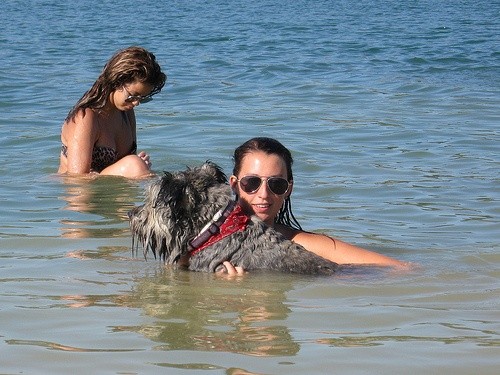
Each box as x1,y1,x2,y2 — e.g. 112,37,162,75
126,159,342,277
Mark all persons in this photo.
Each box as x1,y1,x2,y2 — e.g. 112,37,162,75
54,45,168,177
213,137,409,280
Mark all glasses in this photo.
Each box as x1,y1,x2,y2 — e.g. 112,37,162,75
120,83,153,104
237,176,292,195
